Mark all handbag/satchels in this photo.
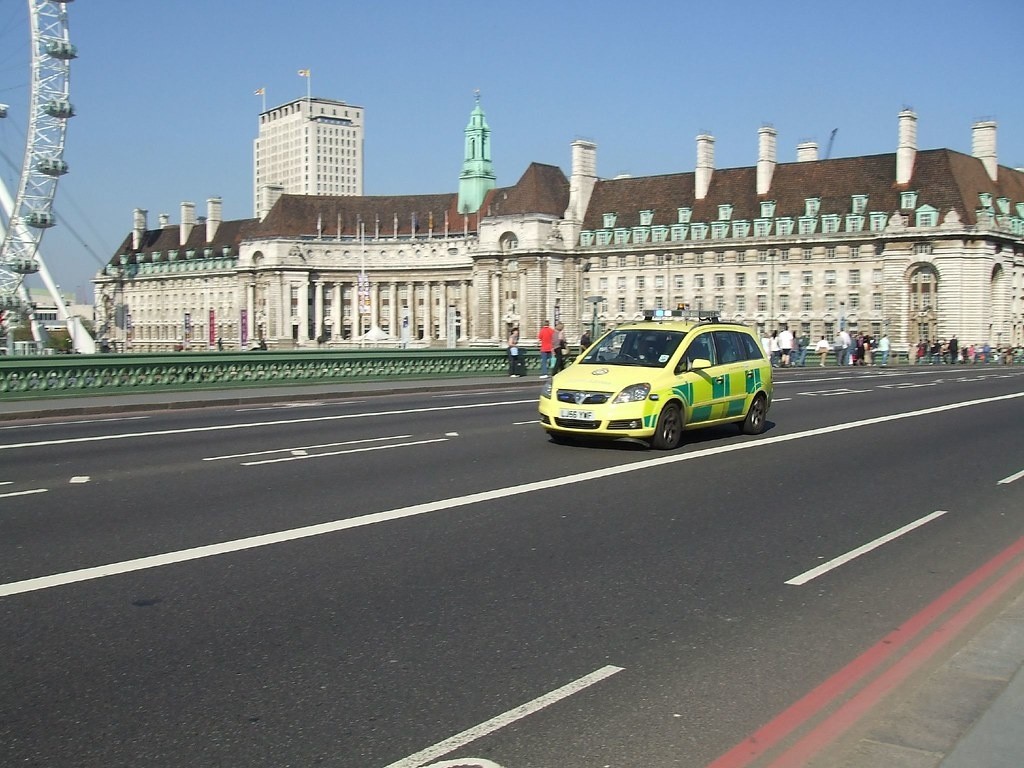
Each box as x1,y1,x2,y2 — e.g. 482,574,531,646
510,347,518,356
560,342,571,355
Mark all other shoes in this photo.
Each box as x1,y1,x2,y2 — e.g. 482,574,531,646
882,363,887,366
539,374,550,378
510,374,520,378
819,364,825,367
772,362,790,368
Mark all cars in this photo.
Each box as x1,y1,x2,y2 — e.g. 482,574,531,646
537,301,774,451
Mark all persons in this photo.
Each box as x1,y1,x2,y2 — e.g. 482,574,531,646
506,328,521,378
539,318,1024,379
